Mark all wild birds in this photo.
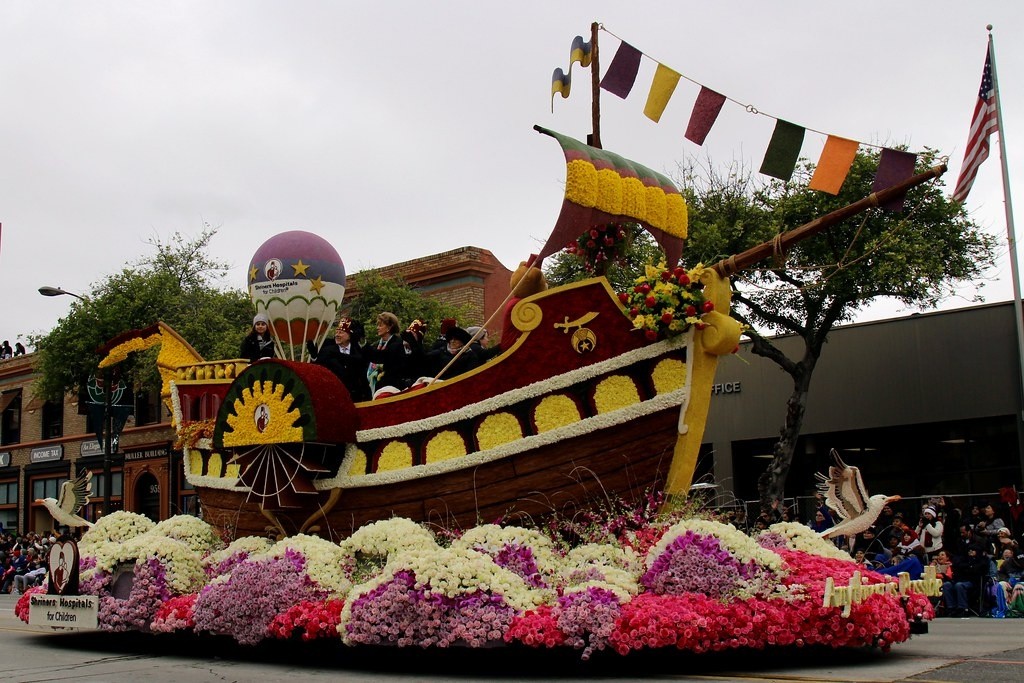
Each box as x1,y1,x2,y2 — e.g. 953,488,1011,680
34,467,95,539
814,449,903,554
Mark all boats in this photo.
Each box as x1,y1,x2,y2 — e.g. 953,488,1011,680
85,19,950,552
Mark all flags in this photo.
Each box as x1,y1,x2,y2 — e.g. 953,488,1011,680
951,38,1007,208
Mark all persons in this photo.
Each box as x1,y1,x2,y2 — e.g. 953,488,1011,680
711,499,833,536
400,317,429,389
848,497,1024,617
1,340,26,358
306,317,367,402
0,522,86,594
430,319,501,379
240,313,275,360
368,312,403,397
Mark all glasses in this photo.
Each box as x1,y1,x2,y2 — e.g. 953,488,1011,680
760,514,768,519
736,509,744,513
815,513,823,518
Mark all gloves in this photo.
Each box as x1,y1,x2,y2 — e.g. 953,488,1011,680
307,340,319,362
350,319,366,340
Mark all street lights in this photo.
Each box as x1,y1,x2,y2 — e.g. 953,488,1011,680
38,285,113,517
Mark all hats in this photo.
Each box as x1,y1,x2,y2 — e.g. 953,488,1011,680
445,326,473,346
405,318,426,341
336,316,354,335
252,313,269,329
439,317,459,335
466,326,487,342
997,526,1012,537
923,504,937,519
48,537,57,542
